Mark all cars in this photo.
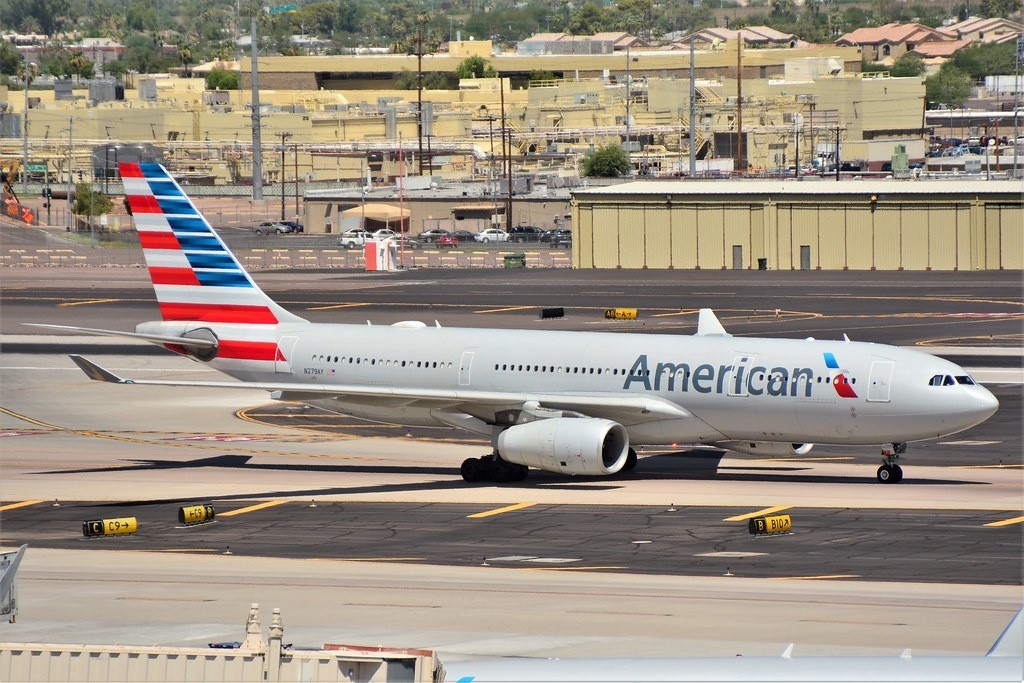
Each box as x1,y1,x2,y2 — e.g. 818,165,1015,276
371,229,403,241
339,232,385,250
417,228,449,244
280,222,303,232
509,225,547,243
540,229,572,249
340,228,371,235
435,234,458,248
785,159,865,176
450,230,476,244
381,234,422,249
252,221,292,235
928,135,1024,156
474,228,508,243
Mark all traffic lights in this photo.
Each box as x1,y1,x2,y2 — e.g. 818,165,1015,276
41,188,45,197
47,188,52,198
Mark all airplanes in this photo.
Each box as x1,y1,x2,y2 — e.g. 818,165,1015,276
20,160,1000,487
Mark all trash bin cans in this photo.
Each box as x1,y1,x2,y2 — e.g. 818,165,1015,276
758,258,767,270
503,253,526,267
